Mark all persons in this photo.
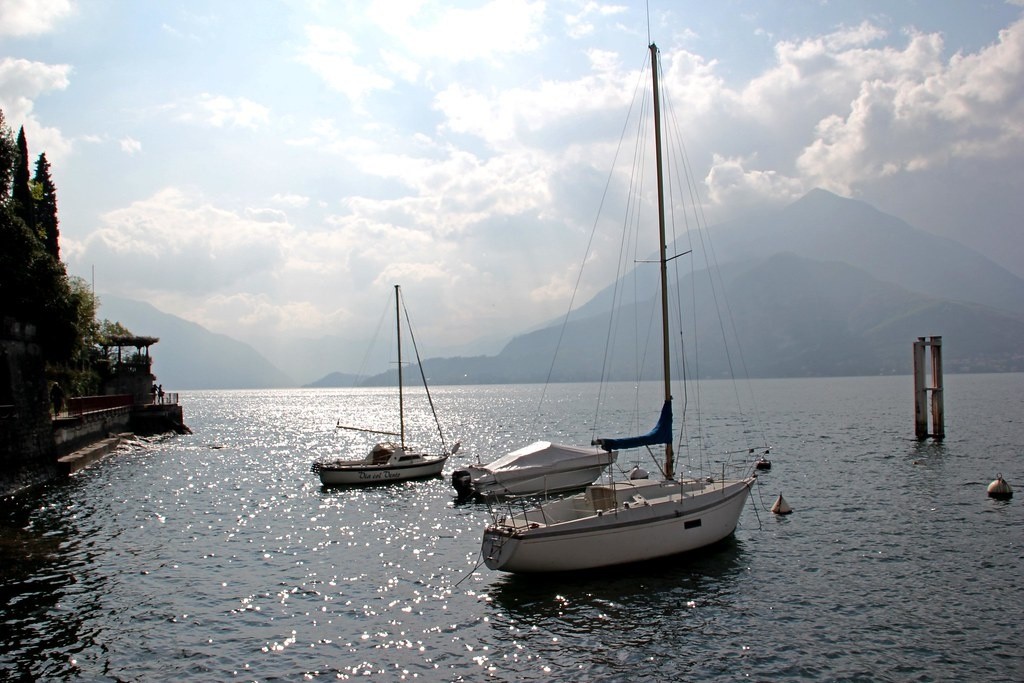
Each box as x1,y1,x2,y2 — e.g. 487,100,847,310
49,381,65,416
16,381,32,401
152,385,157,399
158,384,164,402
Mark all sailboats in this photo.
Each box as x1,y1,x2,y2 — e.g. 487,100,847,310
311,282,461,488
481,42,754,573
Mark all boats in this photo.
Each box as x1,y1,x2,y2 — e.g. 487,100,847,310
452,440,619,499
